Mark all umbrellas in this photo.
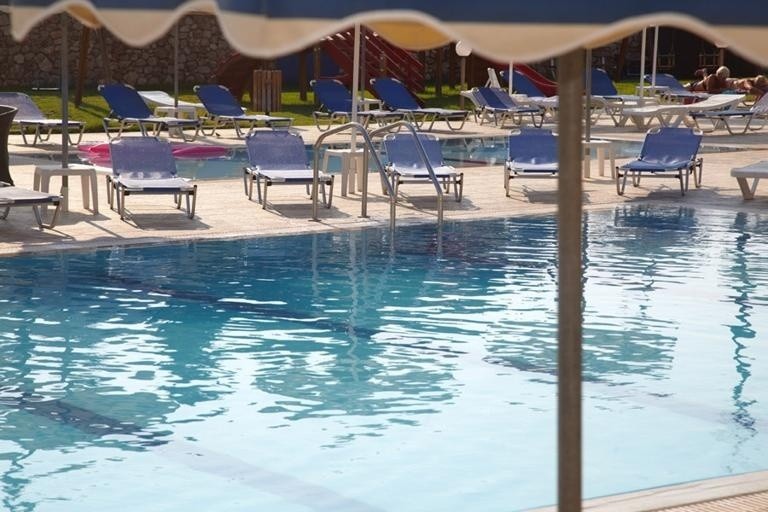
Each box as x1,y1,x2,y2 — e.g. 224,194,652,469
1,0,768,511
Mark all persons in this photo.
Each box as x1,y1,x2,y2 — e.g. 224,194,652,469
683,65,733,106
696,67,768,94
